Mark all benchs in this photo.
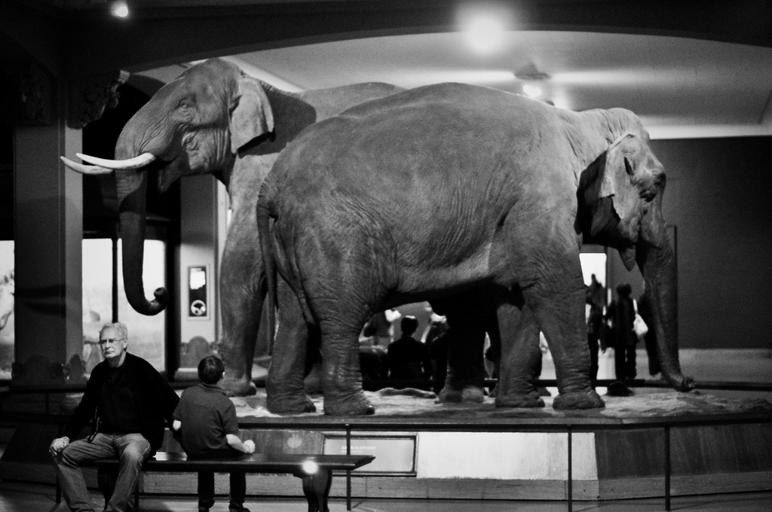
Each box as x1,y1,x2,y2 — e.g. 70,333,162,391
55,451,376,512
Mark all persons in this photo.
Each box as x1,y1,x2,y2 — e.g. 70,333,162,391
49,322,182,512
172,354,255,512
365,269,657,390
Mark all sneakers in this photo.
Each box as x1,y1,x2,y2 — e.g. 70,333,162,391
229,502,249,512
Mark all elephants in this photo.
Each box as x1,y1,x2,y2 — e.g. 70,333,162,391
255,80,696,417
60,57,550,398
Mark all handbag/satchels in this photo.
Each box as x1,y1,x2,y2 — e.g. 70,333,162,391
632,312,649,340
